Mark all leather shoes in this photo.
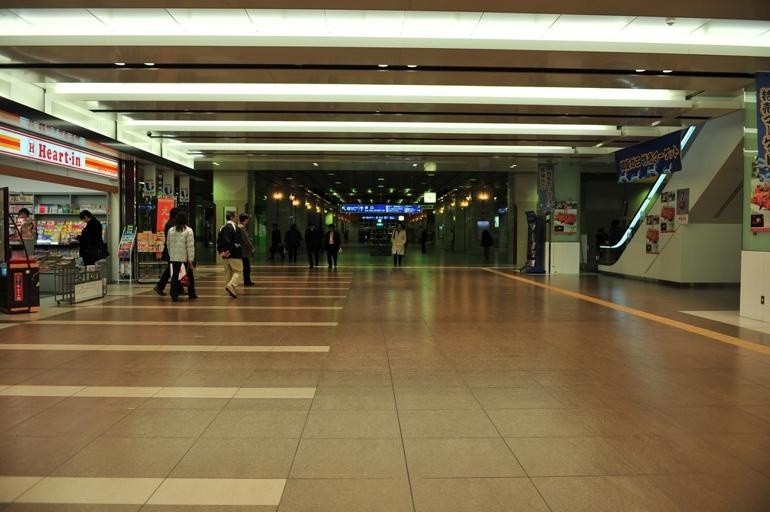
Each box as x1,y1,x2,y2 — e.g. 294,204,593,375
179,292,188,295
172,296,178,302
189,295,197,299
153,286,167,295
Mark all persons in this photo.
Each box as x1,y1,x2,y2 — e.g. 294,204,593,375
645,189,686,252
165,211,198,301
18,207,30,218
154,208,180,296
324,223,342,269
73,210,110,266
761,184,770,200
178,204,188,212
480,228,494,262
268,223,286,264
390,221,406,268
285,223,303,264
216,211,243,298
238,213,255,285
420,228,429,253
305,222,322,269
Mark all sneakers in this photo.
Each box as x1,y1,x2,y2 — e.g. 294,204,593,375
225,284,238,299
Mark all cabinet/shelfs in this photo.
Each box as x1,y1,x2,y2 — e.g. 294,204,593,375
117,224,172,285
9,190,111,293
